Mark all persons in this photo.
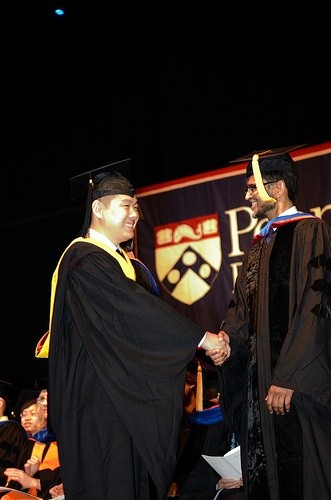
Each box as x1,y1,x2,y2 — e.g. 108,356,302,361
203,145,331,500
0,379,66,500
169,353,247,500
46,157,231,500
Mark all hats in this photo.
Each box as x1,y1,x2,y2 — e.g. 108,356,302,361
65,157,135,202
229,143,306,203
34,377,48,398
13,389,43,415
0,380,15,403
188,351,224,413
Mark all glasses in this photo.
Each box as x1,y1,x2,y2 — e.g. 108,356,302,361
243,181,278,193
19,410,41,419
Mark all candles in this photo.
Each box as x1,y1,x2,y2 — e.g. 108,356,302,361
196,365,204,412
